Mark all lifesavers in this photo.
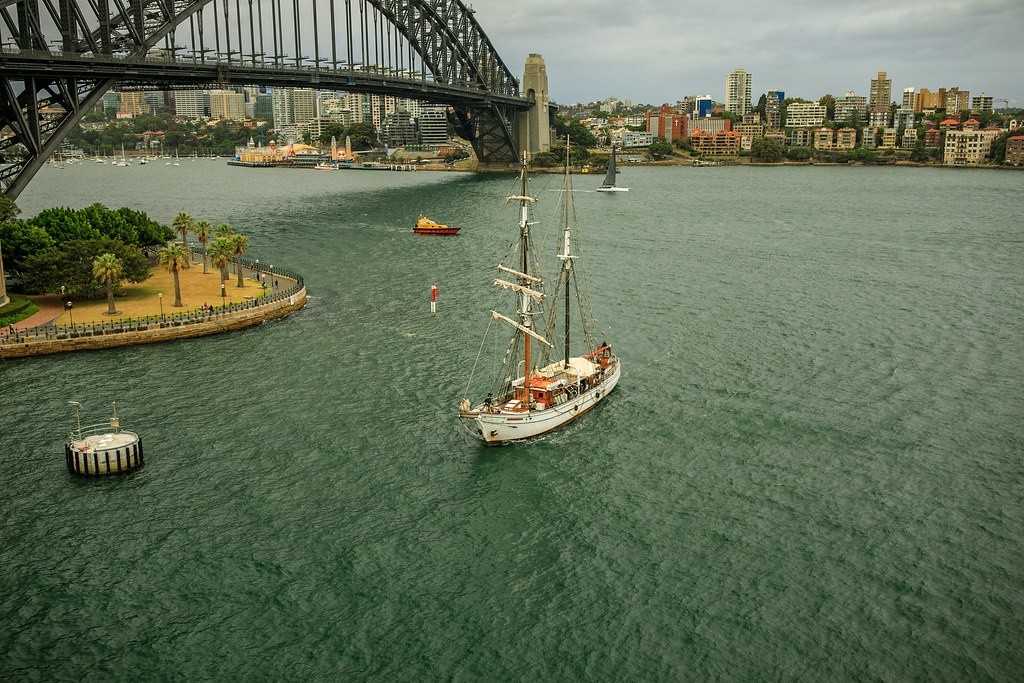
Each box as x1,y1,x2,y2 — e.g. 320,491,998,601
596,393,599,398
574,405,578,410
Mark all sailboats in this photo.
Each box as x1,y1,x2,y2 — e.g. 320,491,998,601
454,133,622,446
46,141,222,170
596,145,629,194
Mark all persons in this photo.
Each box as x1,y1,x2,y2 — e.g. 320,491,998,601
248,261,255,274
274,279,280,290
203,301,214,315
256,273,260,282
8,321,16,334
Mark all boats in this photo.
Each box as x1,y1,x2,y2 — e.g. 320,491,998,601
314,164,340,171
412,226,461,236
